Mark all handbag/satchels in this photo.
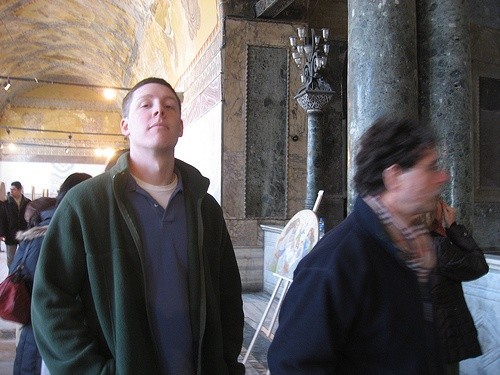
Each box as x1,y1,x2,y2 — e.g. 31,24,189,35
0,238,30,324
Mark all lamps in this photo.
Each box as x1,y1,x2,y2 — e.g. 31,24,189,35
4,80,11,91
289,26,330,82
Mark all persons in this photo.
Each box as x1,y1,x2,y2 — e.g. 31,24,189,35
412,193,490,375
0,181,32,271
29,76,246,375
7,172,93,375
266,115,462,375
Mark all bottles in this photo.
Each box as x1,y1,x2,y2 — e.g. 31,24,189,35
319,218,325,240
